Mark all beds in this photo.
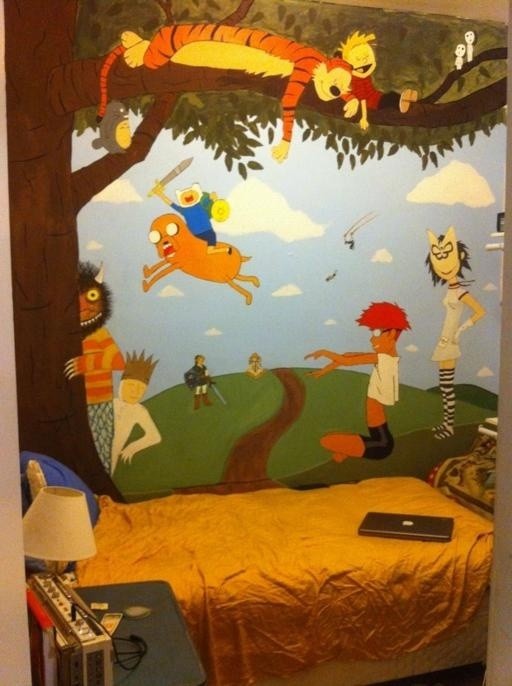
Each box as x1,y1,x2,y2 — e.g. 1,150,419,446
73,476,495,686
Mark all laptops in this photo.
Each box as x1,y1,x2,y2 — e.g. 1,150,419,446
359,513,454,542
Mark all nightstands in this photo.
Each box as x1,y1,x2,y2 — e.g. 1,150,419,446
71,581,207,686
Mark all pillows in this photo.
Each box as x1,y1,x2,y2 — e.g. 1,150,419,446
20,449,101,529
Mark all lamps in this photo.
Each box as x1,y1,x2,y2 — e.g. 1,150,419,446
21,486,98,574
